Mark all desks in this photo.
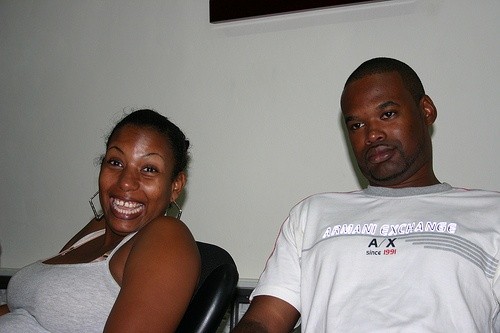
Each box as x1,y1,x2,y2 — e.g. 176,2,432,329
1,266,258,333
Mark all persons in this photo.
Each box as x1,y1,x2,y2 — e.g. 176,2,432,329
0,109,203,333
229,57,500,333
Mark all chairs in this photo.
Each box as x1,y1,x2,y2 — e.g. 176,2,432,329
175,240,239,333
